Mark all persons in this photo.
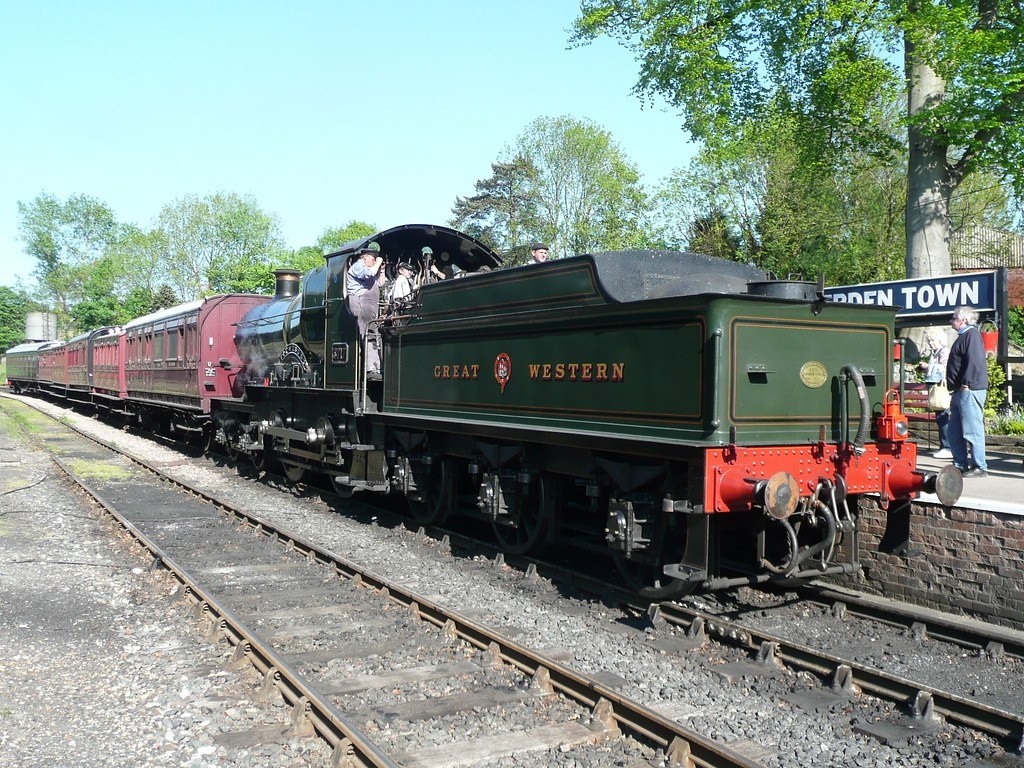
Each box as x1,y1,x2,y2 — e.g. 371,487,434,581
946,305,990,478
478,265,491,273
919,327,956,459
417,246,446,287
451,265,467,279
524,243,549,266
377,262,414,326
347,248,386,380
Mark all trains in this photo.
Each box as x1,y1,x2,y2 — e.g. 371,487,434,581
6,224,963,601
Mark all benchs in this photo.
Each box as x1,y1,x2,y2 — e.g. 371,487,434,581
893,382,937,451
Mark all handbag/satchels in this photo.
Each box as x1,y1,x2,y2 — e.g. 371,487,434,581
928,378,952,410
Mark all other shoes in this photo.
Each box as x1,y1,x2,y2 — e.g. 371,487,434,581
933,448,954,459
367,372,383,381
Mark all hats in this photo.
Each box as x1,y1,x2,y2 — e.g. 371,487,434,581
398,262,413,270
532,243,549,250
361,249,378,258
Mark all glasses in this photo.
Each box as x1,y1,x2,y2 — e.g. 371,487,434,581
952,317,958,321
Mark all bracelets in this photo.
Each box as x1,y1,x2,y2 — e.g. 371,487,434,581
962,385,969,388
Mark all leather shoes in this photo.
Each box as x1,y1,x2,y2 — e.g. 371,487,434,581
963,467,988,477
